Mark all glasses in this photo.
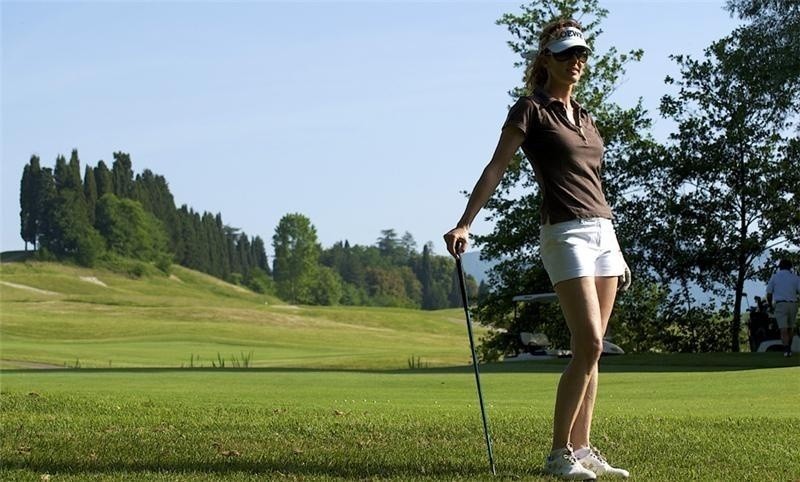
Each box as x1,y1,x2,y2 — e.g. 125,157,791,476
545,46,588,64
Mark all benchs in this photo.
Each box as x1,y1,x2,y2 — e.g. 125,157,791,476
511,330,556,358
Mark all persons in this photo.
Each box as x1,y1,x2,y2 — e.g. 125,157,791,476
765,259,799,358
441,17,630,481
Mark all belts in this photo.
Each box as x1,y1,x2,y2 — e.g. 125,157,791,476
776,298,798,305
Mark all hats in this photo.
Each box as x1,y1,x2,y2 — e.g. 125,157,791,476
537,26,593,57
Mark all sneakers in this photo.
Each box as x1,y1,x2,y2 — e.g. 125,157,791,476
576,442,630,479
783,349,794,361
544,442,598,481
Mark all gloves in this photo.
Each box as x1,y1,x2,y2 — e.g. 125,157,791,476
617,261,632,292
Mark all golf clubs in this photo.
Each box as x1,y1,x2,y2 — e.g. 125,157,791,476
454,241,521,481
